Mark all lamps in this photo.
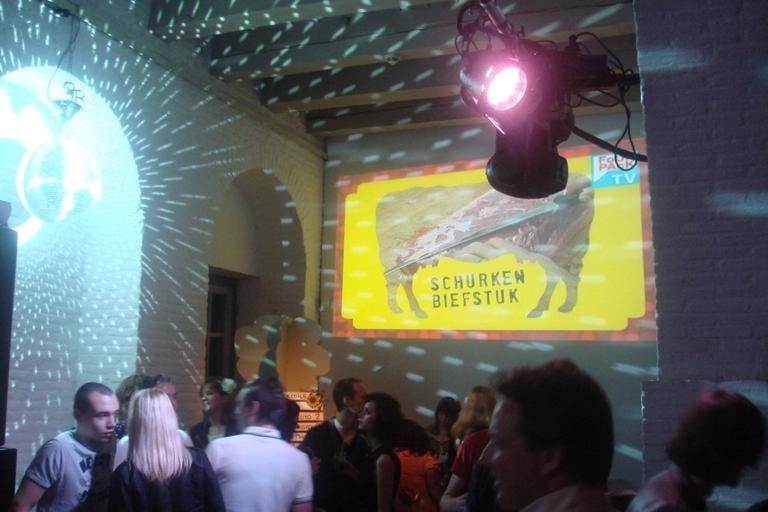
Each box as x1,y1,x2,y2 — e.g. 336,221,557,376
453,2,648,199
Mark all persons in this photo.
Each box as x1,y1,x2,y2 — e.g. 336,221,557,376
298,378,496,512
116,373,300,447
628,391,766,511
204,380,314,511
478,359,637,511
71,388,226,510
15,382,120,510
